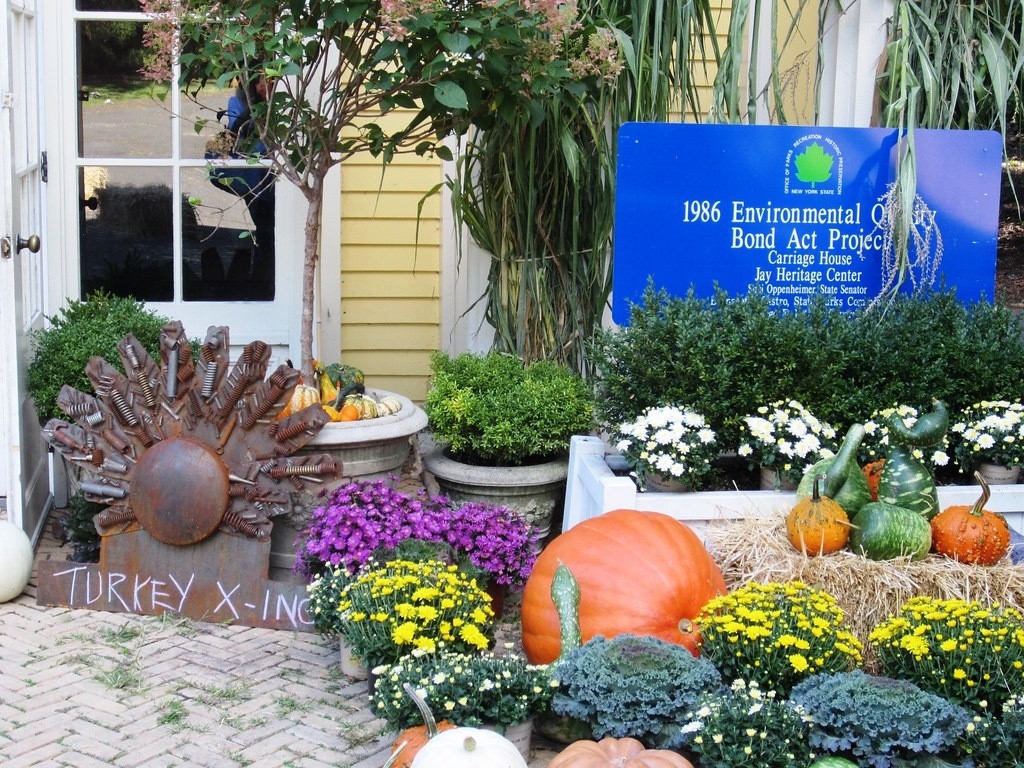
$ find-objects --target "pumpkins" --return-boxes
[388,683,693,768]
[785,459,1011,568]
[274,358,401,421]
[521,510,729,661]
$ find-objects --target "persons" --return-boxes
[227,52,274,232]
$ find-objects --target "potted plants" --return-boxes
[137,0,428,568]
[416,351,600,624]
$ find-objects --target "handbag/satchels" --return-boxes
[204,130,270,197]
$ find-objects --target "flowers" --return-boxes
[294,400,1024,768]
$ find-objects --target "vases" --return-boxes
[480,721,533,763]
[760,467,798,490]
[337,629,369,681]
[977,463,1021,484]
[644,470,695,493]
